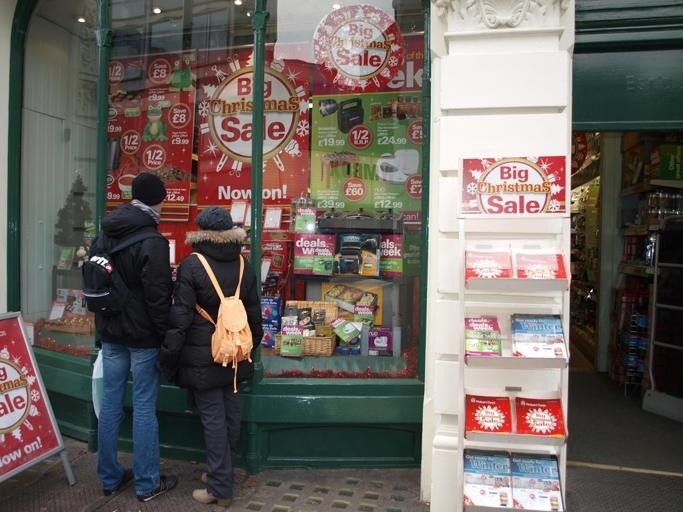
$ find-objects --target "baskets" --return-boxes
[274,299,339,358]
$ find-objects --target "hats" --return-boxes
[130,173,168,207]
[194,206,234,229]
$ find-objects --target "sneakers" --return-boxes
[136,474,179,502]
[102,469,135,496]
[191,471,234,507]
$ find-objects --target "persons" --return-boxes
[86,173,178,501]
[158,205,262,506]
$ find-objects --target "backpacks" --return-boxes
[188,249,253,366]
[80,229,164,319]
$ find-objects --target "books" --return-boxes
[464,242,564,512]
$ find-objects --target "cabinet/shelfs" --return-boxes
[618,177,682,279]
